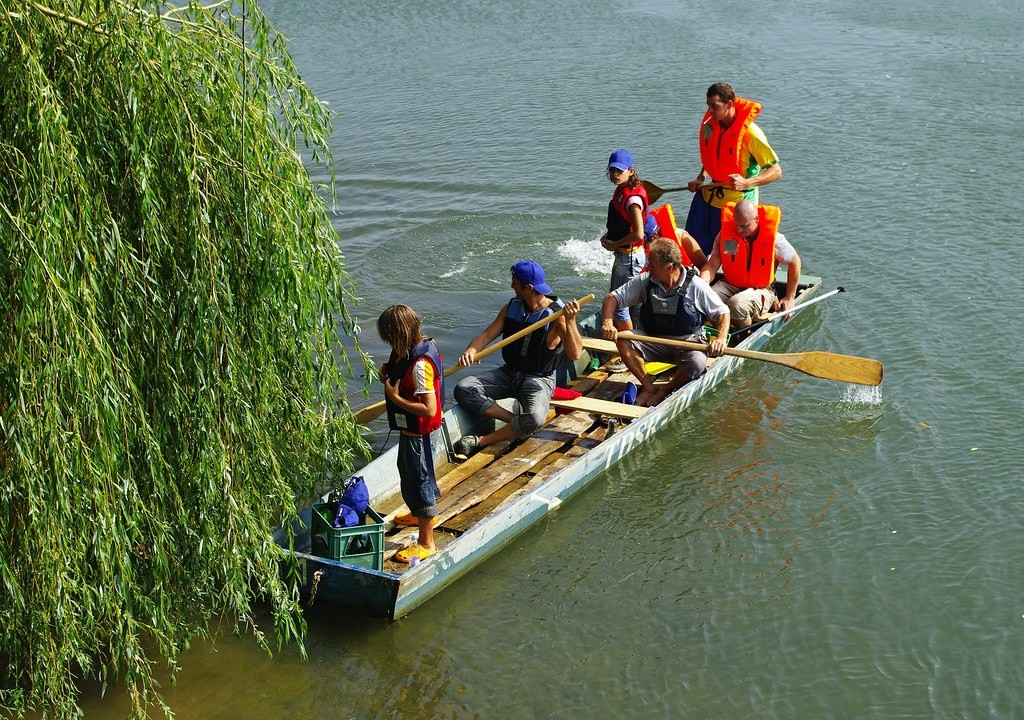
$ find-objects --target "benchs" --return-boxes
[581,336,718,368]
[550,395,650,420]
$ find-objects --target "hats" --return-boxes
[510,260,553,295]
[606,149,633,171]
[644,214,658,243]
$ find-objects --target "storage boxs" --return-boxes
[311,501,385,571]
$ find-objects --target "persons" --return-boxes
[460,260,582,454]
[685,82,782,259]
[601,238,730,406]
[700,200,801,348]
[600,150,648,372]
[641,204,708,276]
[376,304,445,563]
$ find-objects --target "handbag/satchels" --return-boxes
[334,476,369,528]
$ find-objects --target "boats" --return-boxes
[273,267,824,622]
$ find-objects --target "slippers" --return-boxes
[395,544,438,562]
[460,435,479,455]
[394,513,438,526]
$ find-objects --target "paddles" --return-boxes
[700,284,849,348]
[616,332,883,388]
[638,178,736,207]
[344,292,596,427]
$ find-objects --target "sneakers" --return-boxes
[607,358,630,373]
